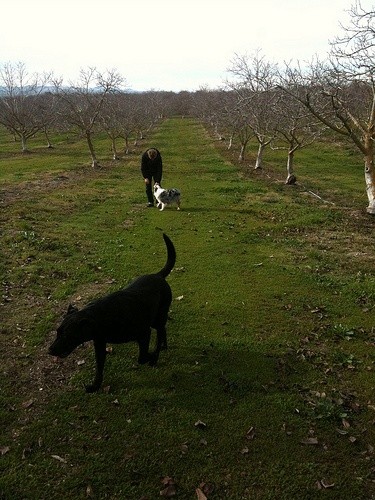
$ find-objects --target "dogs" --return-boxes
[46,233,176,393]
[153,184,181,211]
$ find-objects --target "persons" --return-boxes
[141,148,162,207]
